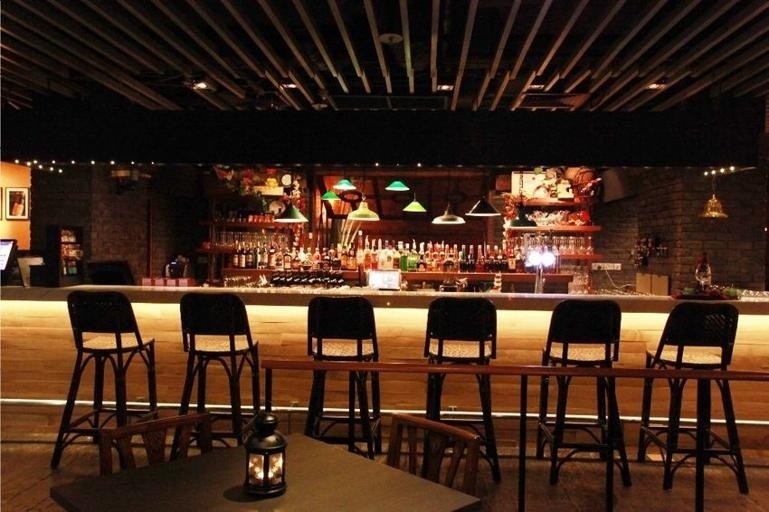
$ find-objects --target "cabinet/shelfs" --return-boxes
[217,265,574,294]
[210,193,314,285]
[44,222,84,285]
[503,195,603,268]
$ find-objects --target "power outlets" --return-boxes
[600,262,608,271]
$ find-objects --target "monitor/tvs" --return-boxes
[0,239,17,272]
[368,269,401,290]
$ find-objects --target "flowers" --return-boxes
[216,166,269,213]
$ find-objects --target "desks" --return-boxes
[47,428,483,511]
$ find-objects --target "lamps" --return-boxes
[240,408,287,496]
[698,168,732,224]
[270,167,543,228]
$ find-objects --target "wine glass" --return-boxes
[522,231,595,256]
[695,263,712,292]
[215,230,287,248]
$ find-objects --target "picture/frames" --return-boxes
[4,185,31,222]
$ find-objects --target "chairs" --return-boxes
[168,290,266,469]
[420,292,507,488]
[635,299,757,511]
[85,407,217,471]
[295,291,385,462]
[89,257,137,285]
[50,287,159,476]
[381,408,484,493]
[535,299,628,493]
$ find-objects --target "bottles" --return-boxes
[270,265,346,287]
[233,240,323,271]
[323,230,515,273]
